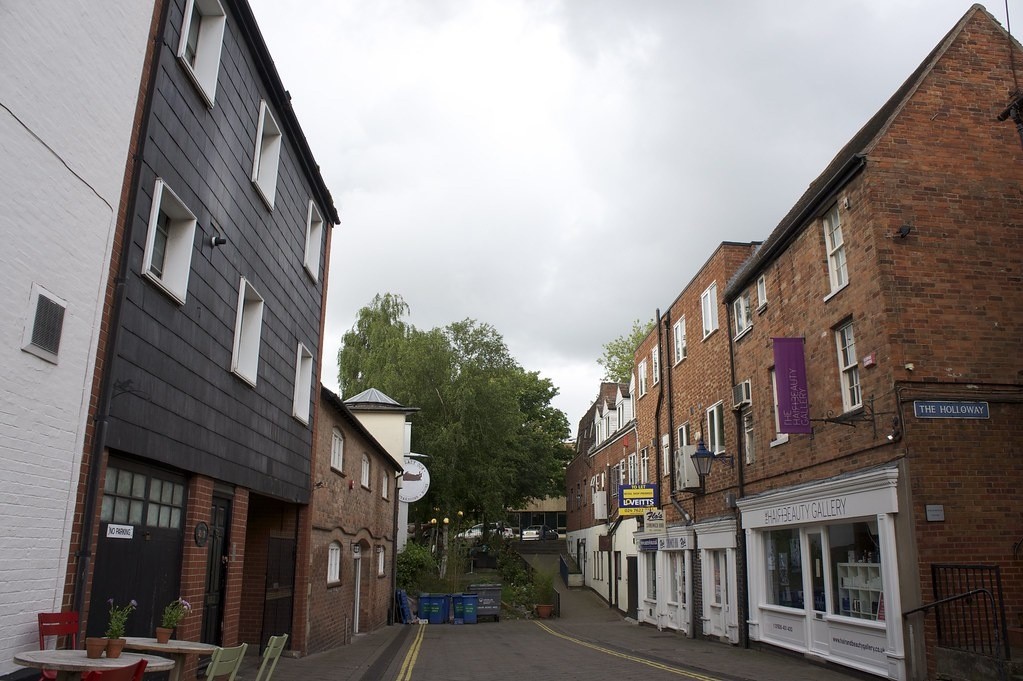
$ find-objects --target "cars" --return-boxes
[522,525,560,540]
[453,523,514,540]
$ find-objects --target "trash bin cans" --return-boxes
[470,583,502,622]
[462,593,478,623]
[429,594,449,624]
[417,593,430,619]
[451,593,464,624]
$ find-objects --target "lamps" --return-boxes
[690,436,734,476]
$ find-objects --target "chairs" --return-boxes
[206,633,289,681]
[37,611,79,681]
[84,658,148,681]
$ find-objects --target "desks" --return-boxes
[13,649,176,681]
[119,637,219,681]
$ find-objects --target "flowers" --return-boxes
[106,598,137,638]
[160,596,192,628]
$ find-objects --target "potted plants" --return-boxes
[534,555,559,618]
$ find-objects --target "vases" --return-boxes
[106,639,126,657]
[155,627,174,644]
[86,638,109,658]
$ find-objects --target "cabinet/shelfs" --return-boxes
[837,562,882,620]
